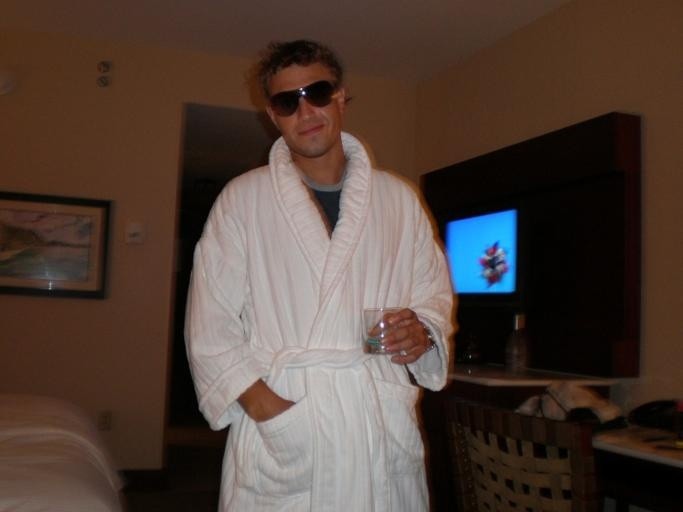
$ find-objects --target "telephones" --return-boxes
[628,398,683,427]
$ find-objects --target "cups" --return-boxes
[362,307,402,356]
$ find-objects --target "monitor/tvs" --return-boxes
[444,207,520,309]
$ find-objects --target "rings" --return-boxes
[398,348,408,358]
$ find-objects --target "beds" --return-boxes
[0,386,122,510]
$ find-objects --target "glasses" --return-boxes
[267,81,340,116]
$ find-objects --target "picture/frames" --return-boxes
[0,191,115,302]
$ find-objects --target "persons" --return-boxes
[184,41,456,511]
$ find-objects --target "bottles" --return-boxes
[507,312,531,377]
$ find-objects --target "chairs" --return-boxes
[445,392,600,512]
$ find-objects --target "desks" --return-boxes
[447,365,683,476]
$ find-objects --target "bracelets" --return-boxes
[424,325,436,354]
